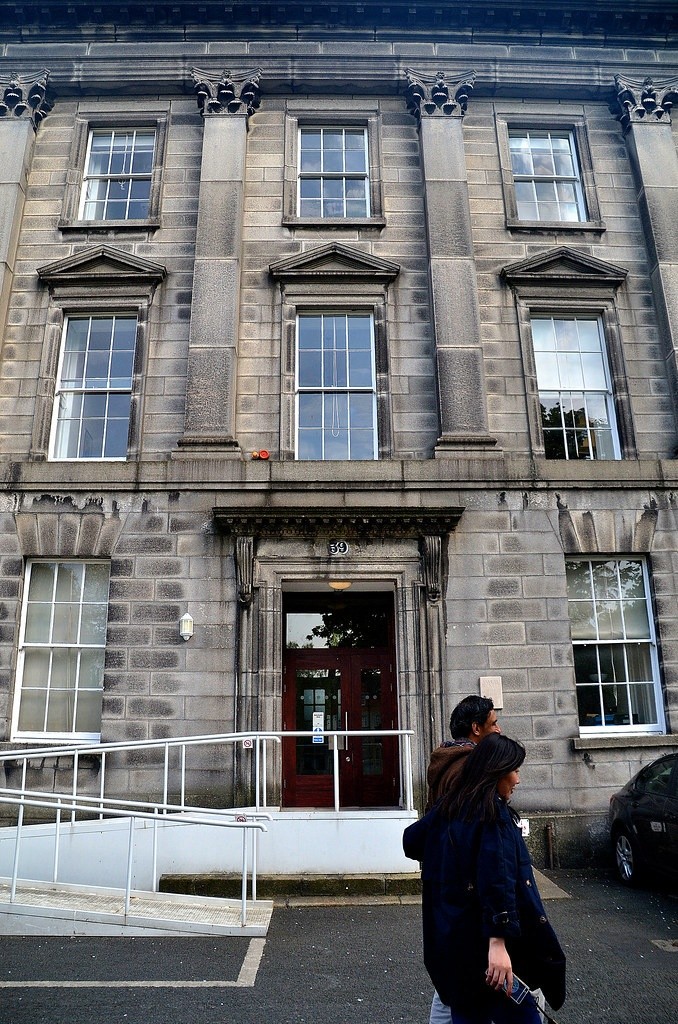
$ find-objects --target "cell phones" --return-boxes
[486,967,530,1005]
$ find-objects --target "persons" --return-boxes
[402,697,566,1024]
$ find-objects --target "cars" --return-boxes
[608,752,678,889]
